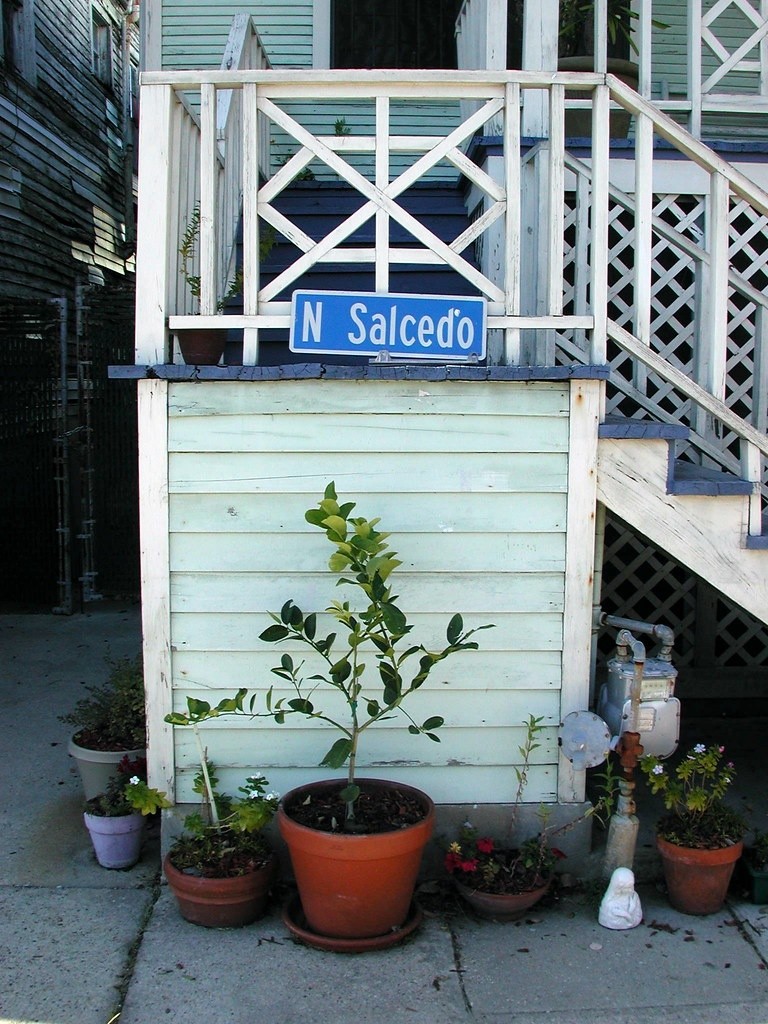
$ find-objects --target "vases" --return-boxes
[655,827,745,915]
[448,869,554,915]
[163,849,282,930]
[84,795,147,870]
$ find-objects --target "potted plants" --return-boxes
[176,199,279,364]
[558,0,671,139]
[736,826,768,905]
[164,481,499,936]
[54,650,146,800]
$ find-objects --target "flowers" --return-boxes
[641,742,752,846]
[126,744,282,875]
[445,711,623,896]
[76,753,147,816]
